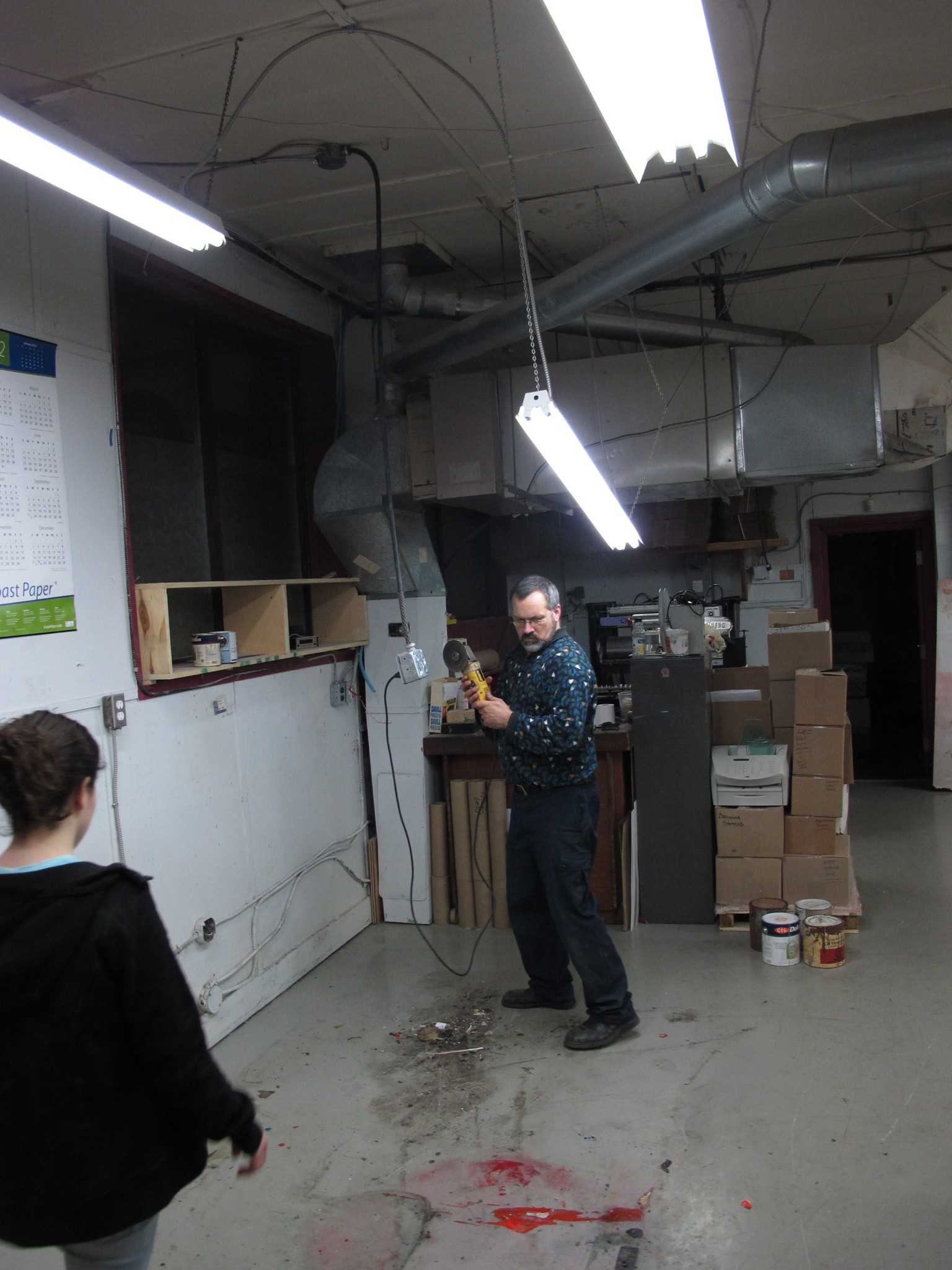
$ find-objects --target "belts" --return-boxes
[514,772,596,795]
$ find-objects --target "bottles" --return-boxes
[631,618,646,658]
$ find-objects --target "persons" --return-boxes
[0,710,268,1270]
[460,576,640,1050]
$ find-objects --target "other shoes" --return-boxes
[501,985,576,1010]
[565,1014,640,1049]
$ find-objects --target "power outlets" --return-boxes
[102,694,127,731]
[330,681,347,706]
[396,648,428,683]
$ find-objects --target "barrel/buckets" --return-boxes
[664,629,689,656]
[749,898,789,952]
[794,898,832,934]
[761,912,801,966]
[802,914,845,968]
[190,630,238,667]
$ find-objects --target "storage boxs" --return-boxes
[710,608,852,909]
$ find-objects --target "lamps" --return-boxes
[0,34,244,253]
[487,0,668,552]
[542,0,739,183]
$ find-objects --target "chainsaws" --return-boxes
[442,639,492,705]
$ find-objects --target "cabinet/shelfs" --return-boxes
[134,576,371,680]
[630,654,718,923]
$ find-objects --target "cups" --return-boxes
[617,691,632,722]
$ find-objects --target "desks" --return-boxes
[423,723,631,918]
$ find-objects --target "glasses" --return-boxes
[512,610,553,627]
